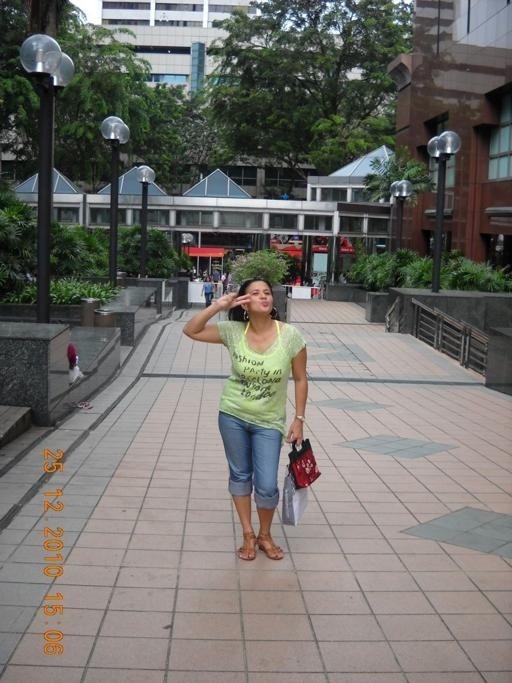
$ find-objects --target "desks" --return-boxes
[100,116,130,289]
[427,130,461,292]
[135,165,156,277]
[390,180,413,251]
[182,233,193,256]
[19,33,76,322]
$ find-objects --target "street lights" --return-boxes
[282,441,308,526]
[288,439,322,490]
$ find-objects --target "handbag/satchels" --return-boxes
[294,415,305,424]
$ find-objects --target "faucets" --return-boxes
[237,530,257,560]
[257,528,285,560]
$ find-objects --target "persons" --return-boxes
[182,277,309,561]
[311,269,351,287]
[61,324,85,385]
[190,266,230,308]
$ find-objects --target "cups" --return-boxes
[240,276,264,293]
